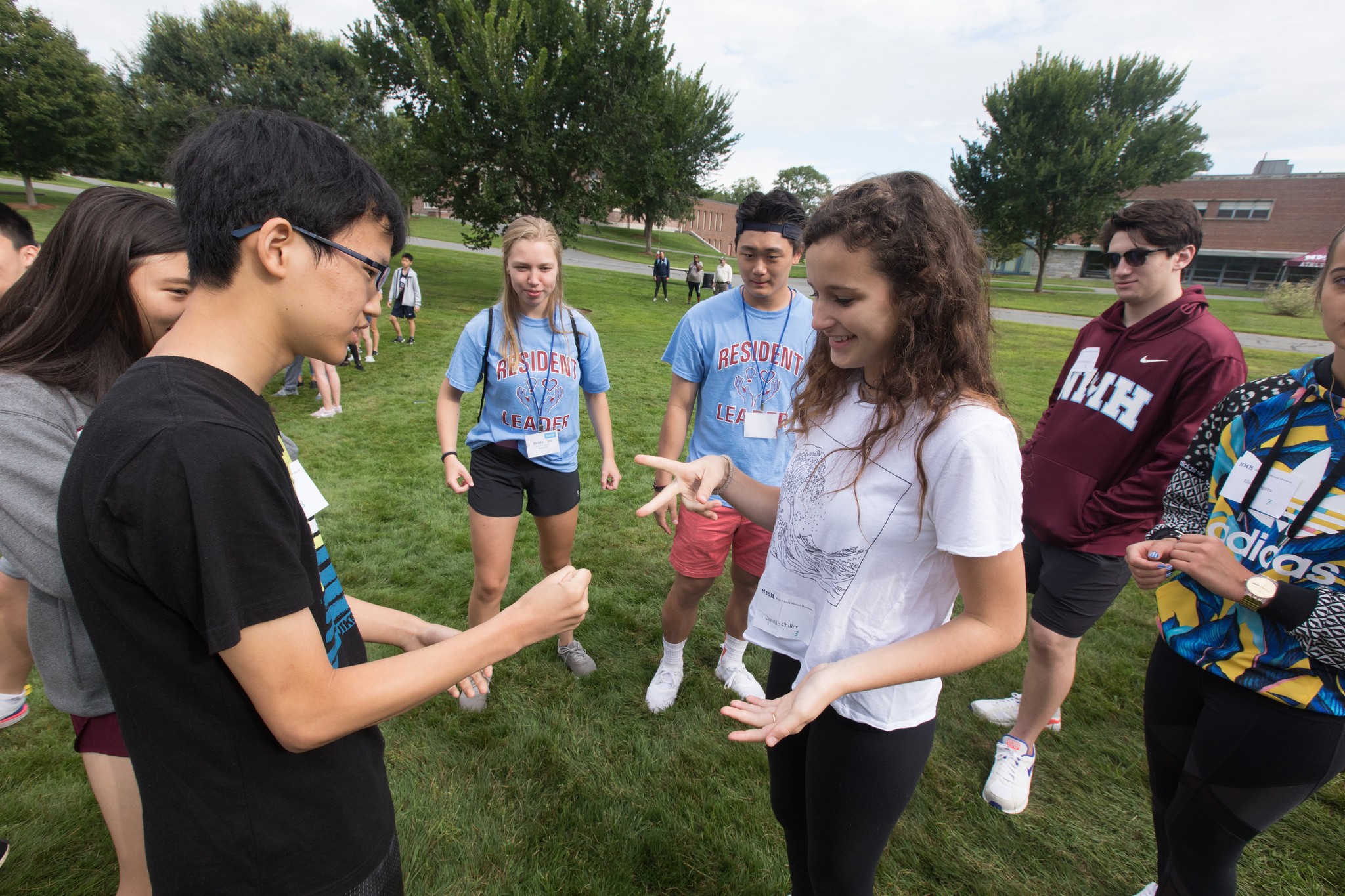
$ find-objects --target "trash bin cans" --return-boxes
[702,272,714,289]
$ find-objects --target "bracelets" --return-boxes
[711,454,734,495]
[441,451,458,463]
[653,482,668,492]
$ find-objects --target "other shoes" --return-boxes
[296,380,305,387]
[0,683,33,729]
[310,381,318,389]
[1133,880,1159,896]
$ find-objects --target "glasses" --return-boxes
[1097,248,1172,270]
[232,220,390,292]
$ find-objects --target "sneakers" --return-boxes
[270,387,298,397]
[333,404,343,415]
[457,672,488,712]
[644,659,684,714]
[714,643,766,703]
[980,729,1036,815]
[339,336,415,372]
[310,406,336,418]
[315,392,322,401]
[556,637,597,679]
[969,691,1061,735]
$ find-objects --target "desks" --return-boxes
[648,265,704,274]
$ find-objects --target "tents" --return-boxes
[1274,246,1329,290]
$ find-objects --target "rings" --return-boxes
[772,713,777,723]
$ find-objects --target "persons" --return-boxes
[970,197,1248,815]
[653,251,670,302]
[1124,223,1345,896]
[0,187,193,895]
[712,257,732,296]
[437,215,621,714]
[57,109,593,896]
[272,254,422,419]
[686,255,703,304]
[636,171,1028,896]
[644,187,821,713]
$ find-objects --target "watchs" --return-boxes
[696,262,698,264]
[1238,573,1278,613]
